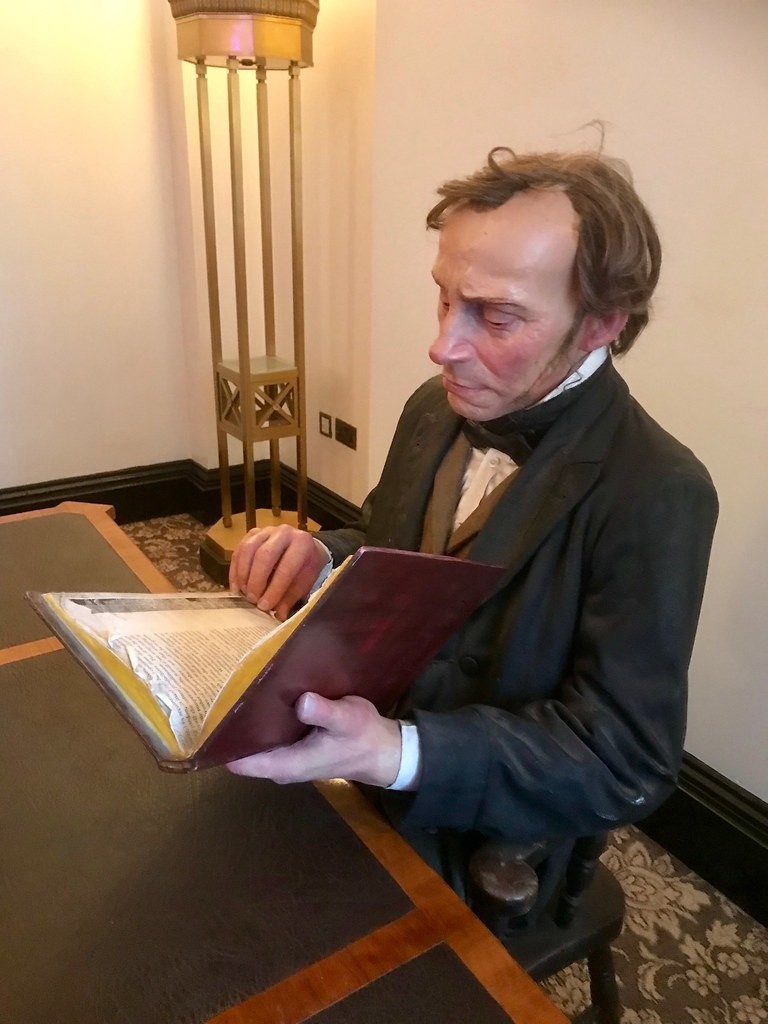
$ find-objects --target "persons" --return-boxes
[227,147,720,938]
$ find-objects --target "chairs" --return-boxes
[457,834,627,1024]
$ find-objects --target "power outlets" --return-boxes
[319,411,333,439]
[335,417,357,451]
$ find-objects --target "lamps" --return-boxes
[169,0,322,594]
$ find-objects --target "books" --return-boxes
[23,548,506,773]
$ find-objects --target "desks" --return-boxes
[0,500,573,1024]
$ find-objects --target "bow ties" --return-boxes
[459,406,540,470]
